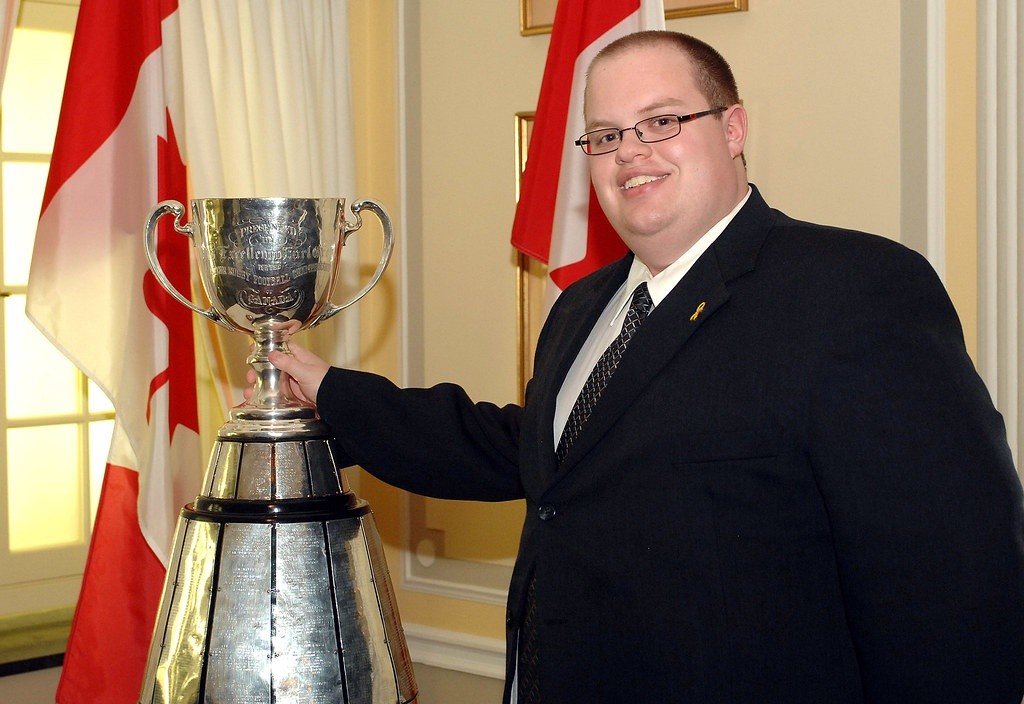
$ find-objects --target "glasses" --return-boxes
[574,107,729,156]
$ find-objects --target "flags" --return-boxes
[510,1,666,291]
[24,2,198,704]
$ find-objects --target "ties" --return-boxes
[517,282,653,704]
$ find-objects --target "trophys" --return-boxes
[143,198,396,437]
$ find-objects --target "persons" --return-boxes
[244,31,1024,703]
